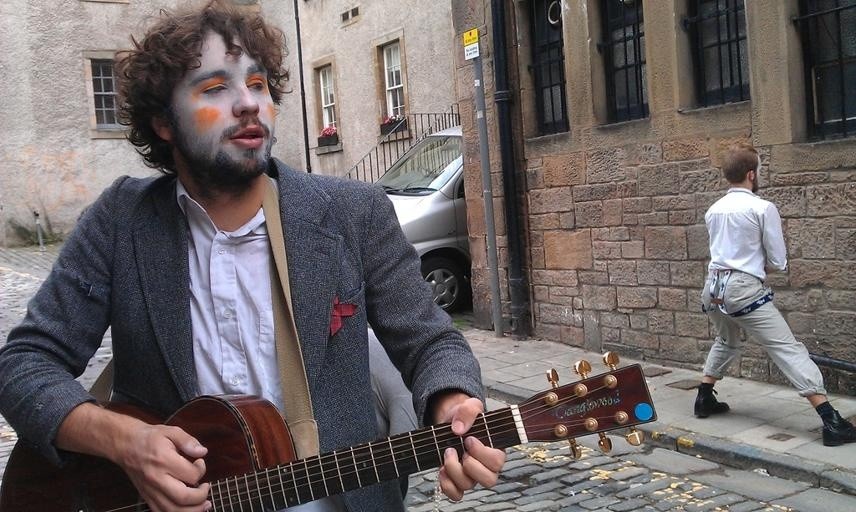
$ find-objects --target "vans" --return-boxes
[372,123,472,317]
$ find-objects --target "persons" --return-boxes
[692,147,856,446]
[0,5,506,511]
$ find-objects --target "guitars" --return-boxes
[0,350,657,512]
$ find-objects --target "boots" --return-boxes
[821,410,855,447]
[692,383,730,418]
